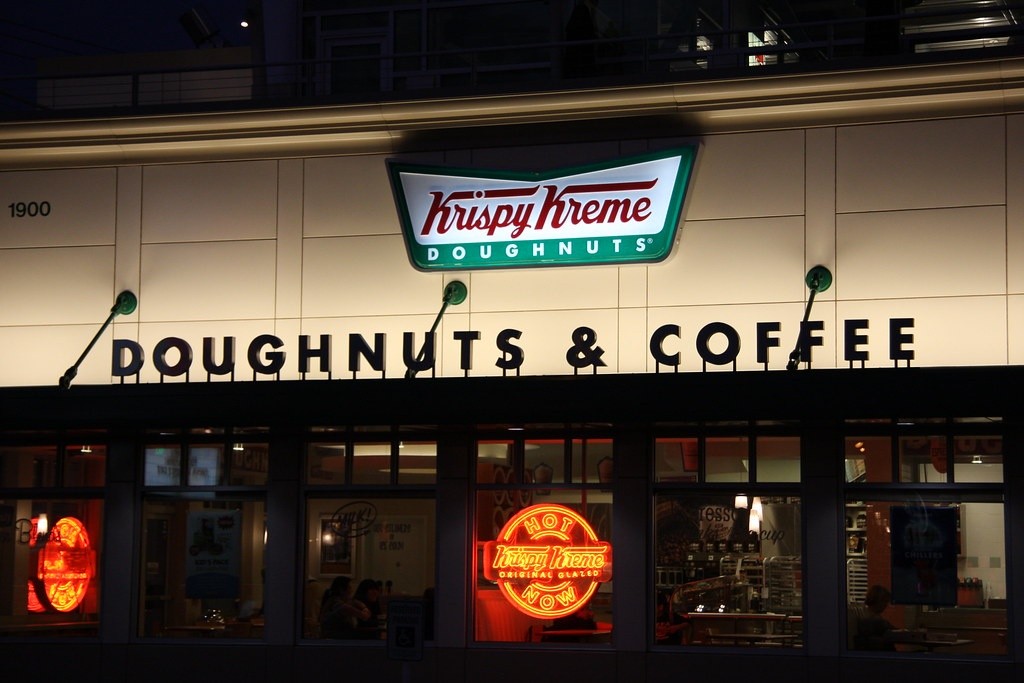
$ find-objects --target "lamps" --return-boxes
[786,265,832,372]
[59,291,137,391]
[404,281,467,380]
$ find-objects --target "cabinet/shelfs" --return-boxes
[846,504,867,556]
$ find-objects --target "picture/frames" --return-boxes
[316,514,356,579]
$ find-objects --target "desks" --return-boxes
[684,612,804,648]
[537,630,612,645]
[165,625,233,639]
[869,636,975,654]
[710,633,799,649]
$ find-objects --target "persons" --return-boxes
[318,576,395,642]
[847,585,896,652]
